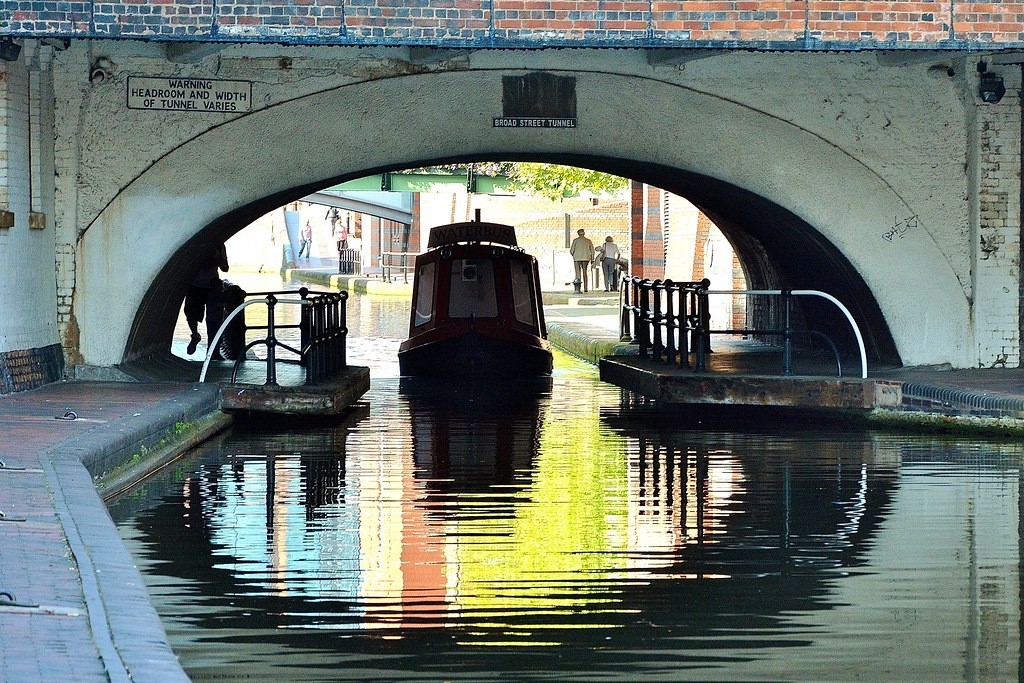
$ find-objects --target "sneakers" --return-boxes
[186,332,201,355]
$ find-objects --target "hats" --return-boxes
[577,228,584,232]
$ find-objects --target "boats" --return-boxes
[396,220,554,377]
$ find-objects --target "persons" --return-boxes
[570,227,594,292]
[334,218,349,256]
[297,218,313,262]
[183,238,231,361]
[324,206,340,237]
[599,236,620,293]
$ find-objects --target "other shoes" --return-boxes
[306,257,309,262]
[298,253,301,257]
